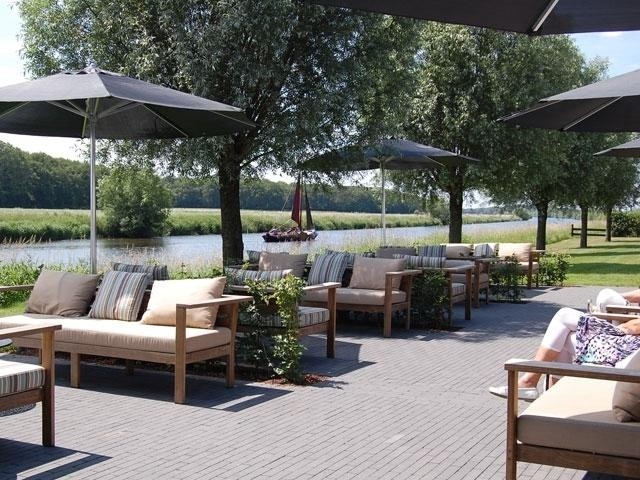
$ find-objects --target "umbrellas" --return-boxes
[294,137,481,248]
[308,0,640,38]
[593,138,640,158]
[495,68,640,133]
[1,63,259,275]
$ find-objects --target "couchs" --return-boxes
[225,281,342,376]
[1,285,254,404]
[300,265,424,337]
[0,322,62,446]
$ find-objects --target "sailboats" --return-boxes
[263,172,318,242]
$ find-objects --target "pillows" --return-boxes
[325,243,532,266]
[25,270,98,318]
[110,261,170,280]
[349,255,407,290]
[139,275,228,329]
[87,269,152,321]
[246,249,289,262]
[259,251,308,281]
[224,268,293,314]
[612,350,640,424]
[307,253,348,285]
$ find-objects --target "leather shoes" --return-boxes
[587,299,592,313]
[488,386,539,402]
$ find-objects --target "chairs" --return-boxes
[504,303,640,480]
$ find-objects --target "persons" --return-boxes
[488,287,640,403]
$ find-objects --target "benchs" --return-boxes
[304,250,544,327]
